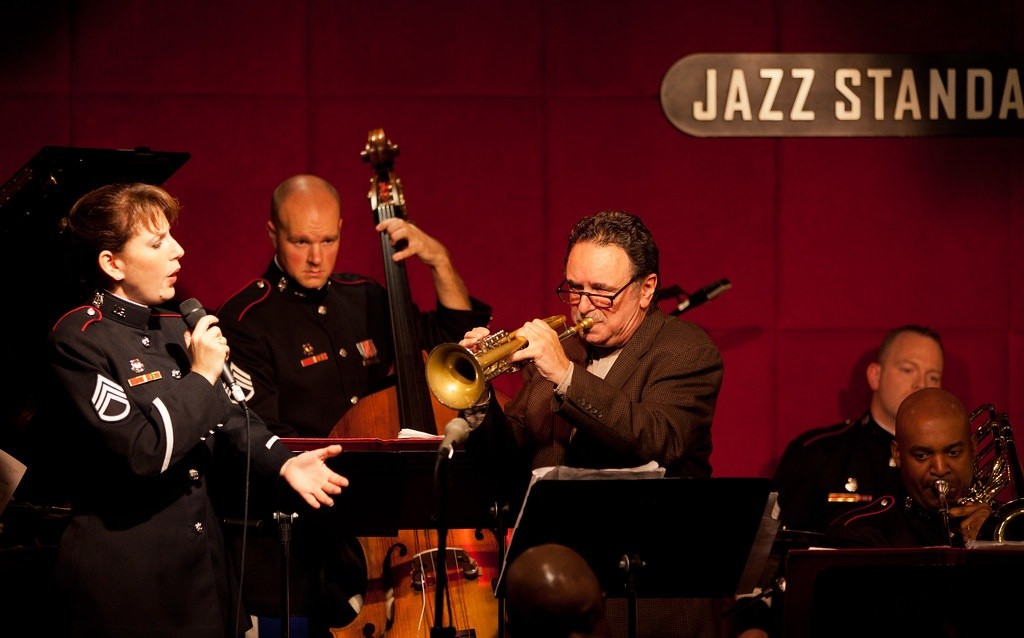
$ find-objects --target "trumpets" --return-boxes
[424,310,593,410]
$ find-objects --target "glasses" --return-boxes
[556,273,643,309]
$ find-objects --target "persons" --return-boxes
[811,388,1024,638]
[36,183,349,638]
[456,212,723,638]
[732,324,945,638]
[201,169,496,638]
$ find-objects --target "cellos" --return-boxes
[328,127,503,638]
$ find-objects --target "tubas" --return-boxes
[932,403,1024,550]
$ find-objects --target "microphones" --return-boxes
[179,298,248,411]
[668,279,732,316]
